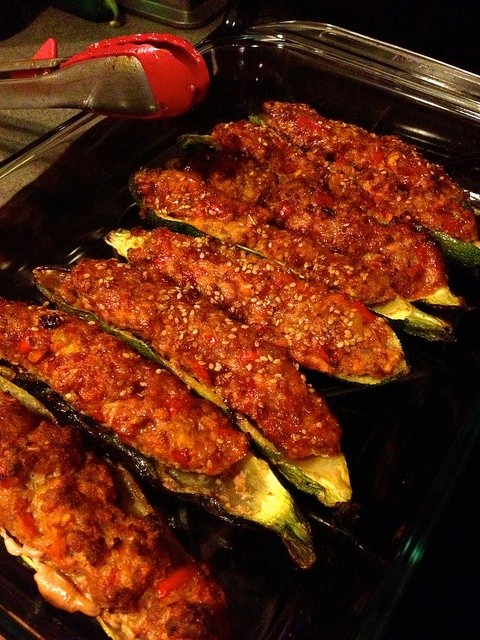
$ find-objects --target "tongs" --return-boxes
[0,32,210,122]
